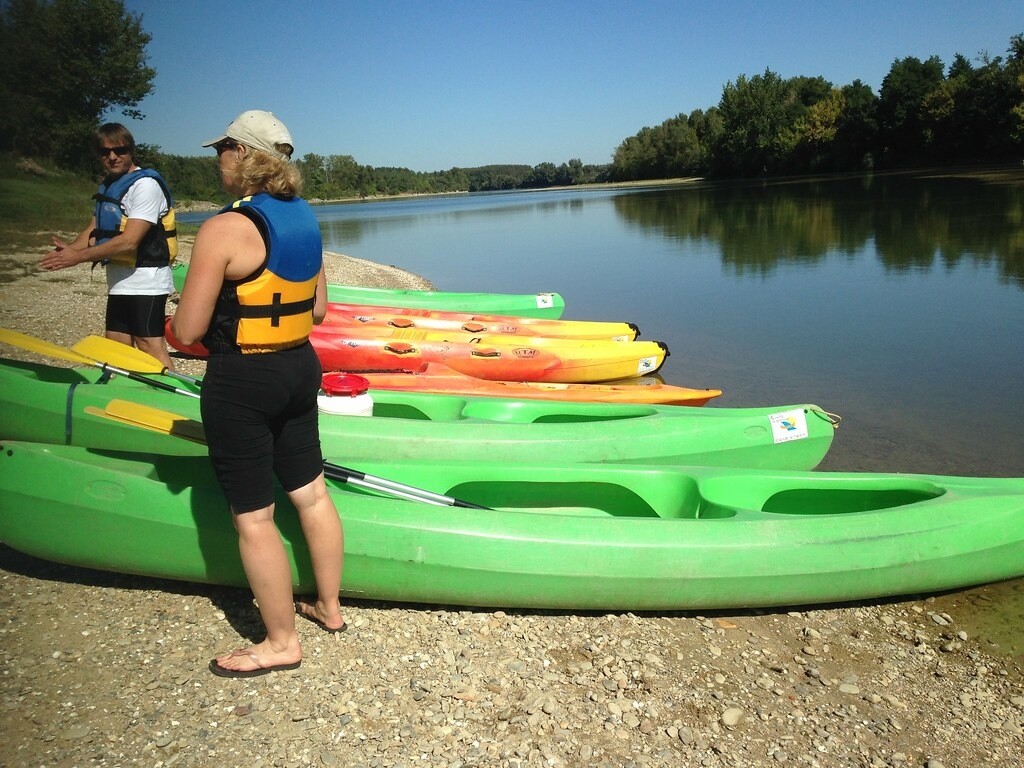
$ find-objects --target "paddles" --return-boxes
[82,406,461,506]
[72,334,202,389]
[106,399,496,510]
[1,325,204,399]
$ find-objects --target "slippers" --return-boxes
[295,597,348,634]
[208,649,301,678]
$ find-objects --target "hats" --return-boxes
[203,109,294,164]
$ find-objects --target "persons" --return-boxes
[170,109,347,679]
[39,122,178,371]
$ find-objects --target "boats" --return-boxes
[320,302,640,339]
[326,373,721,406]
[0,440,1022,612]
[324,282,567,320]
[308,327,672,383]
[1,360,836,471]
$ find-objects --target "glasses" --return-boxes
[99,146,133,155]
[216,144,242,156]
